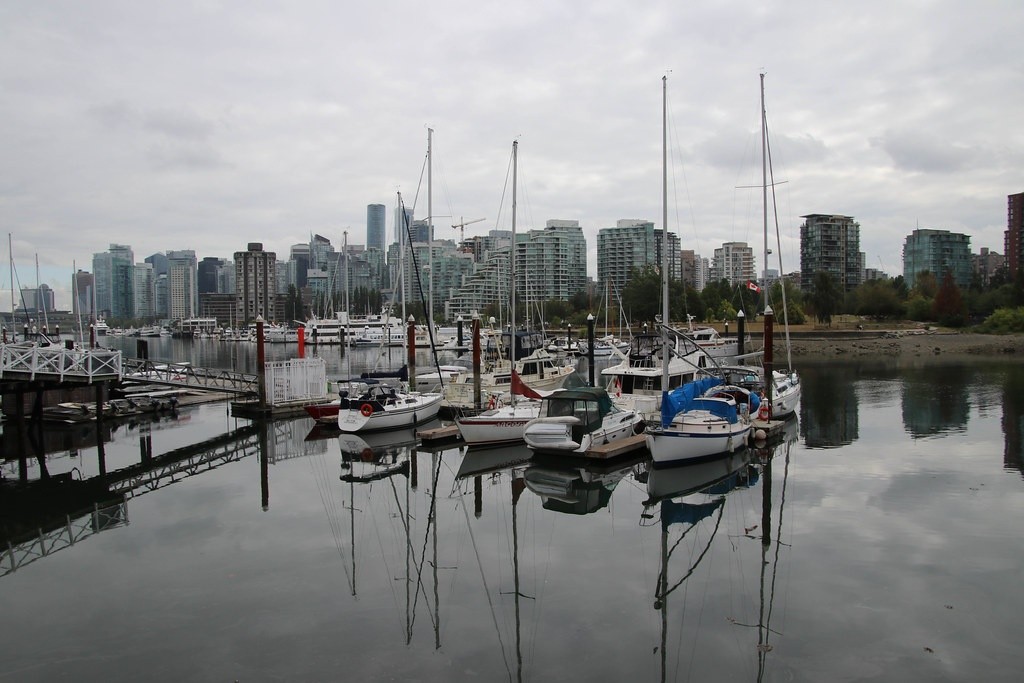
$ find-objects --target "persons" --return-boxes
[488,394,496,409]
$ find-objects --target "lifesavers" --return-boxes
[759,406,773,421]
[361,403,373,417]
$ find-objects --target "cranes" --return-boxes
[452,216,487,247]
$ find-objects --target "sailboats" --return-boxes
[0,65,804,471]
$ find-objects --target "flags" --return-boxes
[747,279,760,293]
[510,369,542,399]
